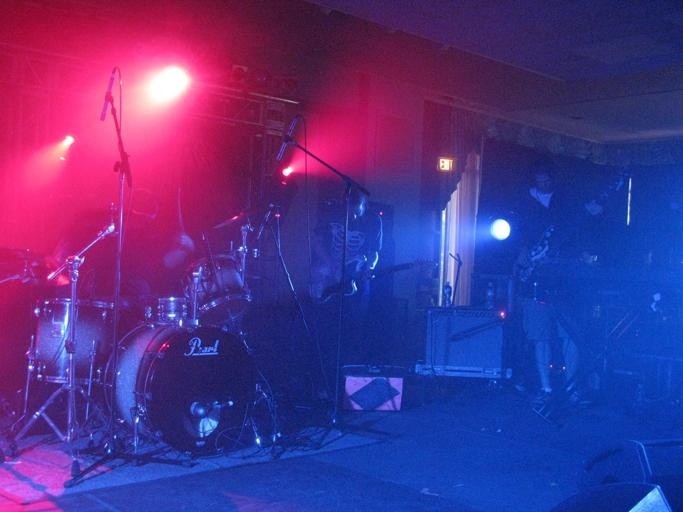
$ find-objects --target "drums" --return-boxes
[35,298,129,389]
[157,296,189,327]
[179,253,252,326]
[103,321,254,456]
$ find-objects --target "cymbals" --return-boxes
[211,204,268,230]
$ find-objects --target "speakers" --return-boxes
[555,478,675,512]
[423,307,510,379]
[603,434,680,483]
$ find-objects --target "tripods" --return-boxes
[5,240,116,477]
[294,142,390,454]
[68,98,196,486]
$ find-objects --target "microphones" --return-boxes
[248,204,276,251]
[277,121,291,164]
[99,76,115,123]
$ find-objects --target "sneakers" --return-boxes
[529,387,593,411]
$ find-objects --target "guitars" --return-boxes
[310,253,438,302]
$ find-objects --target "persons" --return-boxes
[312,189,386,367]
[498,162,612,412]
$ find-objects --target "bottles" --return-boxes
[484,281,494,311]
[442,281,452,309]
